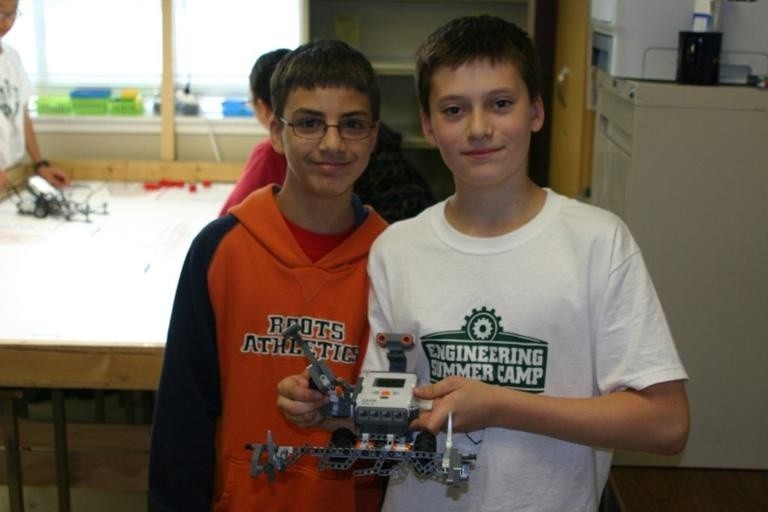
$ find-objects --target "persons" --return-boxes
[0,0,70,201]
[219,50,291,218]
[278,14,690,512]
[150,39,391,512]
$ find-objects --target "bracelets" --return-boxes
[33,159,50,169]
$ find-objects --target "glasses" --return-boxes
[277,116,379,141]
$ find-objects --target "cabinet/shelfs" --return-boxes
[301,0,538,209]
